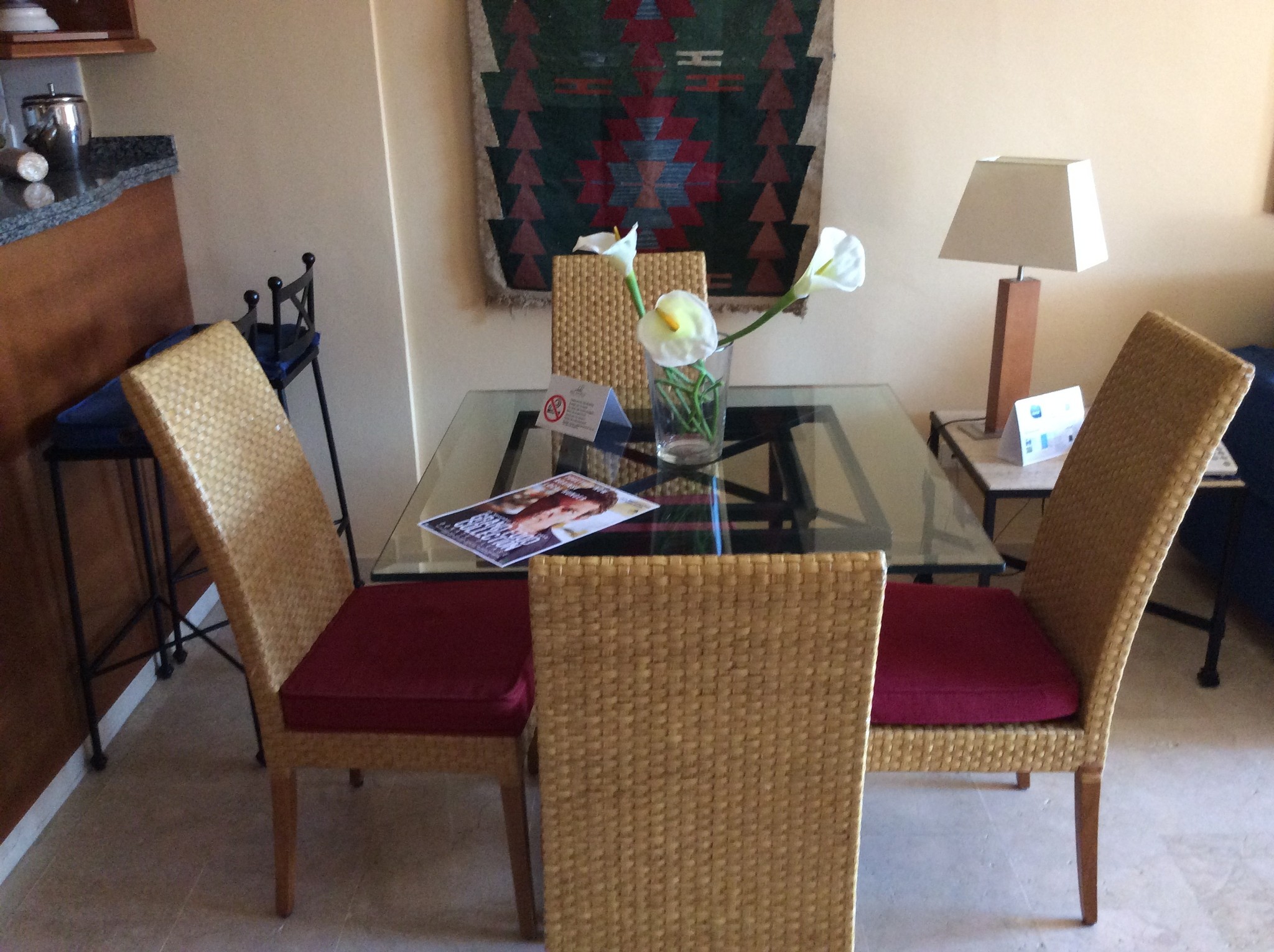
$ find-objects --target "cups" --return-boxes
[0,146,49,182]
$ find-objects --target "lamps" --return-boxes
[943,155,1109,439]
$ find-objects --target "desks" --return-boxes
[927,408,1249,686]
[373,387,1010,587]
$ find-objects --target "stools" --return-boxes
[40,255,359,771]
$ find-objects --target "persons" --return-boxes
[430,486,618,565]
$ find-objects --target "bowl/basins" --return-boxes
[1,8,60,33]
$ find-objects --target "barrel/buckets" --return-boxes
[22,83,91,160]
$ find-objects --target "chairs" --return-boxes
[552,250,733,531]
[863,308,1256,924]
[131,316,540,937]
[519,544,893,952]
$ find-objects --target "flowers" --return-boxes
[572,222,866,439]
